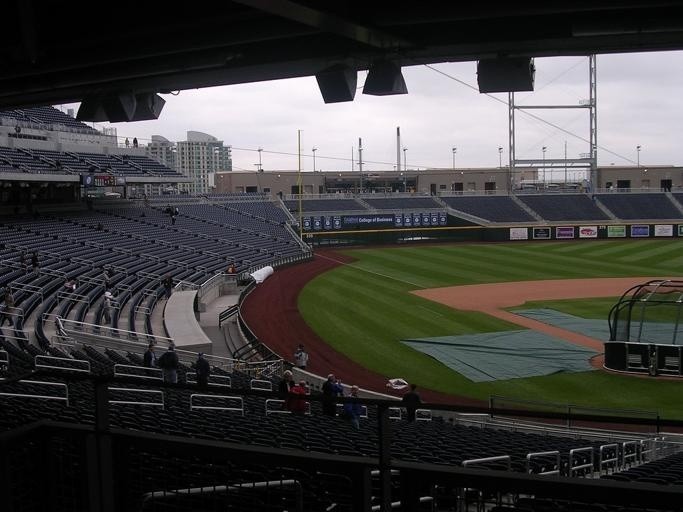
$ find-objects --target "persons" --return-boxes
[125,138,130,148]
[56,158,62,169]
[279,190,282,200]
[166,203,179,225]
[133,138,138,149]
[0,240,421,430]
